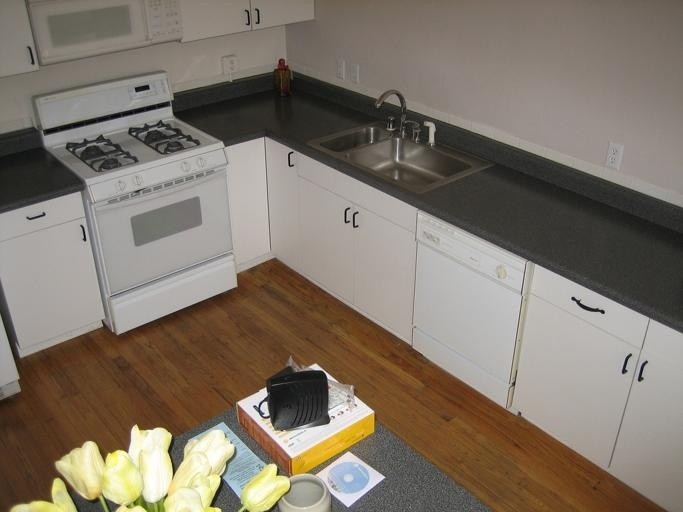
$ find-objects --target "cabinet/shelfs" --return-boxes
[176,0,315,44]
[0,0,40,79]
[0,192,105,359]
[299,176,413,347]
[265,138,299,275]
[511,264,683,512]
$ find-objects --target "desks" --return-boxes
[47,407,495,512]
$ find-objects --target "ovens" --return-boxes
[84,141,238,337]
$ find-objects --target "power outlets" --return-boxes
[605,141,624,170]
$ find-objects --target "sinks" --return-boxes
[305,122,401,155]
[345,132,496,194]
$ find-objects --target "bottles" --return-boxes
[273,58,291,97]
[276,473,332,512]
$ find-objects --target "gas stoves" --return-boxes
[53,117,222,179]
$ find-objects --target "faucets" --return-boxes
[423,121,436,145]
[374,88,407,129]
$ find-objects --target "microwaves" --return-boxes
[27,0,183,67]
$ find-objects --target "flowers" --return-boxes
[9,424,291,512]
